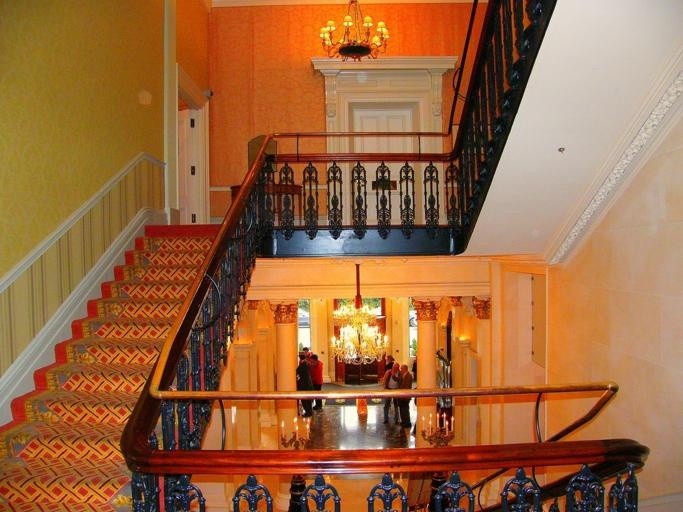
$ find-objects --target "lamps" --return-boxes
[319,0,390,63]
[329,263,389,367]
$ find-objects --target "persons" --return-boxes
[310,354,323,410]
[384,354,394,368]
[380,362,402,424]
[297,351,314,417]
[397,364,412,427]
[298,347,312,364]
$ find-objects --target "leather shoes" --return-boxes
[303,406,321,417]
[383,420,401,425]
